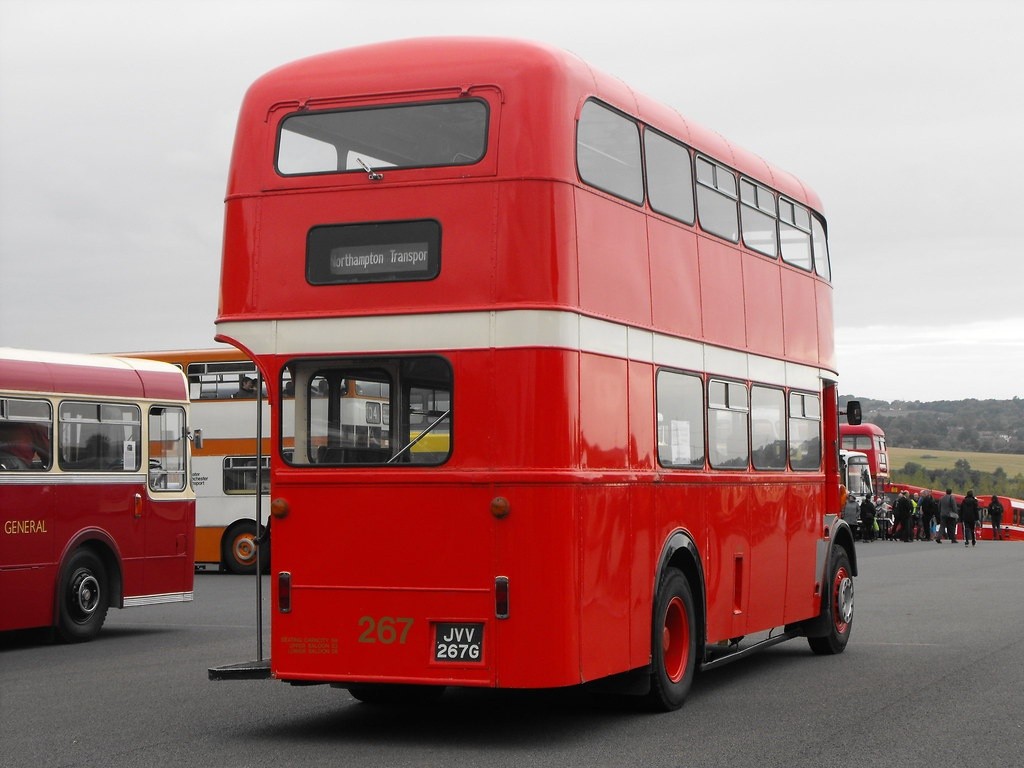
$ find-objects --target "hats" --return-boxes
[850,493,856,500]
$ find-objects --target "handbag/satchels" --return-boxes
[866,513,872,518]
[930,518,937,533]
[873,517,879,532]
[948,512,958,524]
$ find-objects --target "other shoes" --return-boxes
[952,539,958,543]
[965,542,969,546]
[936,539,941,543]
[972,541,976,546]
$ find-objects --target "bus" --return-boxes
[409,390,452,466]
[839,423,1024,540]
[213,34,860,714]
[101,351,391,574]
[0,345,197,636]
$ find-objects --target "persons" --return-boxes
[0,420,51,472]
[283,382,294,398]
[842,487,980,547]
[235,377,259,399]
[986,495,1004,542]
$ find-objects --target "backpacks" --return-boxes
[992,503,1000,514]
[892,501,897,516]
[895,500,906,520]
